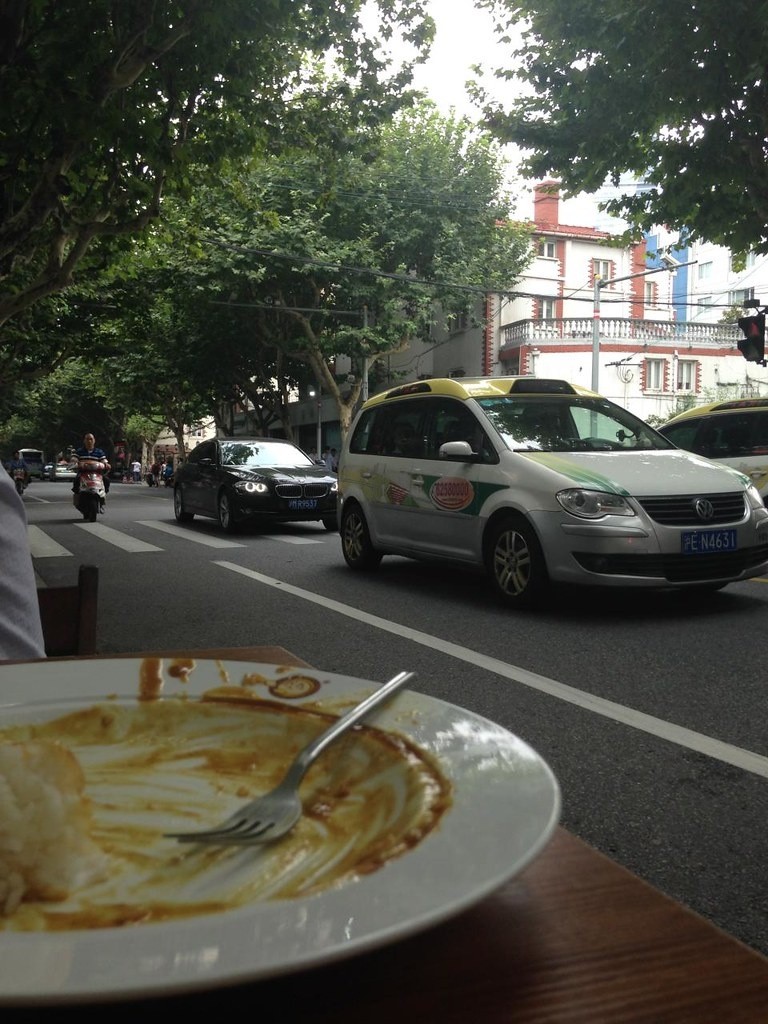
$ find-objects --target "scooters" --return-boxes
[68,448,111,521]
[7,464,30,496]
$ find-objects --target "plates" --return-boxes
[0,657,563,1009]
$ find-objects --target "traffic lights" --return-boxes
[735,313,767,363]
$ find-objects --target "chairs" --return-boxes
[37,563,98,657]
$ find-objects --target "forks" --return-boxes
[161,666,417,850]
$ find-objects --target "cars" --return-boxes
[52,462,78,482]
[335,374,768,608]
[173,437,341,536]
[43,465,53,479]
[614,393,768,512]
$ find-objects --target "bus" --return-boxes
[18,449,44,480]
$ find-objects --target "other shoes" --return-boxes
[100,507,105,514]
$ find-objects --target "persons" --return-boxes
[71,433,112,494]
[308,446,339,472]
[389,423,425,459]
[150,461,174,487]
[132,460,141,480]
[9,451,30,483]
[0,463,48,660]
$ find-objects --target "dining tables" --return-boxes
[0,645,768,1024]
